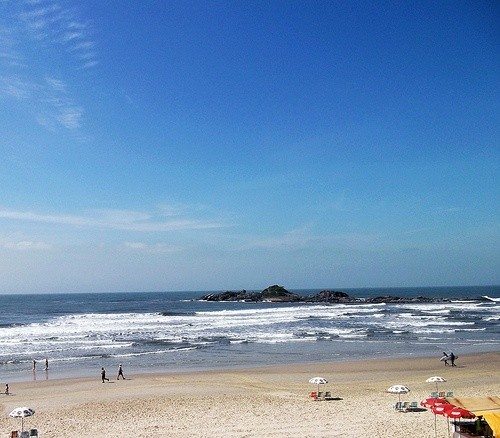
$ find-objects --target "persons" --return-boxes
[32,359,36,371]
[116,364,125,381]
[5,384,9,394]
[44,359,48,370]
[443,352,449,366]
[450,352,457,366]
[101,367,110,383]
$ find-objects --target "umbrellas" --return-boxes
[432,404,456,434]
[443,406,475,431]
[309,377,328,392]
[388,384,411,401]
[425,376,447,393]
[420,398,450,430]
[10,407,36,431]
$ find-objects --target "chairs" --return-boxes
[11,429,38,438]
[396,401,418,412]
[430,391,453,398]
[309,391,331,401]
